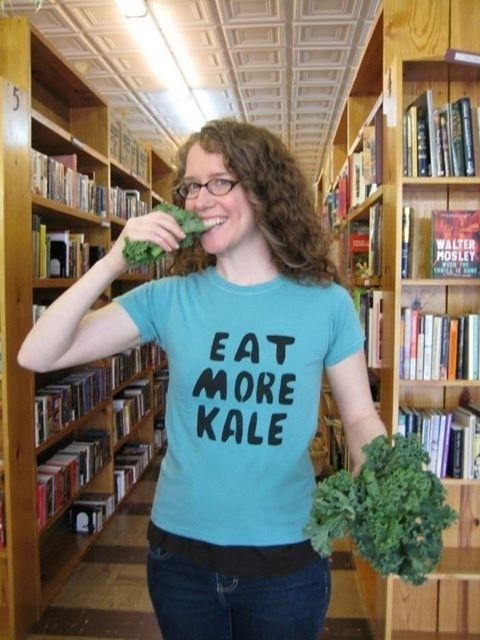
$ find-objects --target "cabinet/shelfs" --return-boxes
[0,13,179,640]
[316,1,480,640]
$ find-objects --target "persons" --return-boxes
[15,117,389,639]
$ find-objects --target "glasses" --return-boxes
[174,178,240,200]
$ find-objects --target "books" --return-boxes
[29,147,169,279]
[32,294,170,534]
[397,405,480,479]
[401,206,480,280]
[399,307,479,381]
[320,285,383,473]
[321,106,383,278]
[403,90,475,178]
